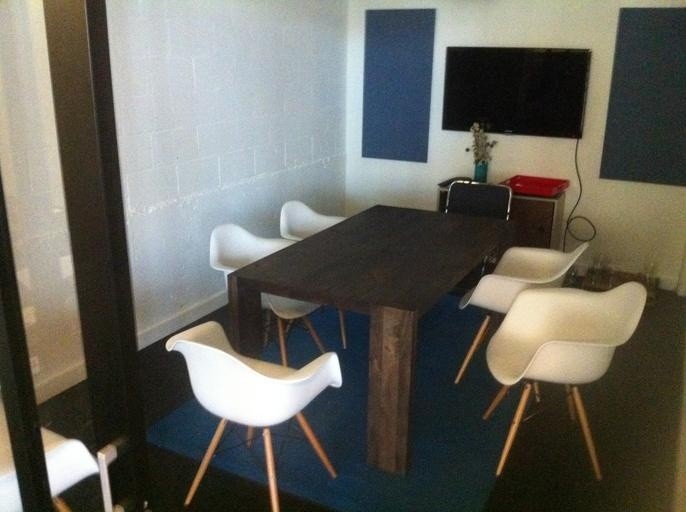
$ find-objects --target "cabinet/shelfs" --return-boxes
[437,175,566,296]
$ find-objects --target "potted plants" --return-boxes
[470,122,499,183]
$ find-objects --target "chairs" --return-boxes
[483,282,648,482]
[278,201,354,353]
[208,222,335,367]
[164,320,344,511]
[436,179,516,289]
[451,238,594,404]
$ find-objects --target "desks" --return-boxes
[0,402,101,511]
[227,204,521,473]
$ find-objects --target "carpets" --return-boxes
[144,282,684,510]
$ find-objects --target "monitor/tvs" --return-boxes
[443,47,592,139]
